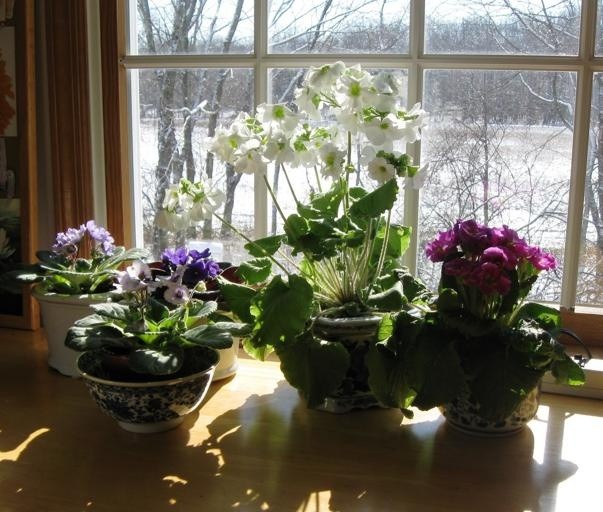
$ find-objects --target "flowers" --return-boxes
[374,218,586,425]
[6,219,150,295]
[152,61,430,420]
[146,246,247,314]
[64,259,254,378]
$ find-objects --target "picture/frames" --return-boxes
[0,0,42,331]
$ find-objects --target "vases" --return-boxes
[75,346,221,432]
[212,314,241,383]
[31,282,124,378]
[437,373,541,439]
[306,304,422,415]
